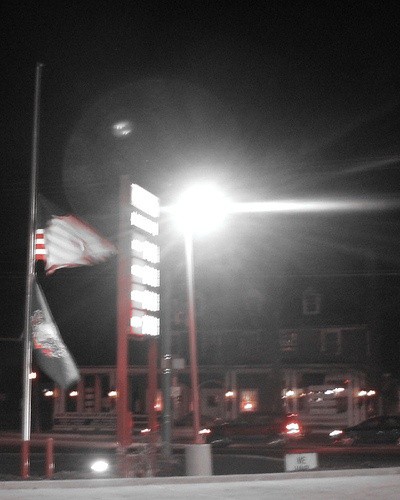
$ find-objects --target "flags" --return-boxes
[32,194,118,276]
[26,282,82,390]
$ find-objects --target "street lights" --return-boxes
[174,188,211,476]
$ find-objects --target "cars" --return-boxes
[199,411,304,449]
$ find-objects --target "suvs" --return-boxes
[330,414,400,448]
